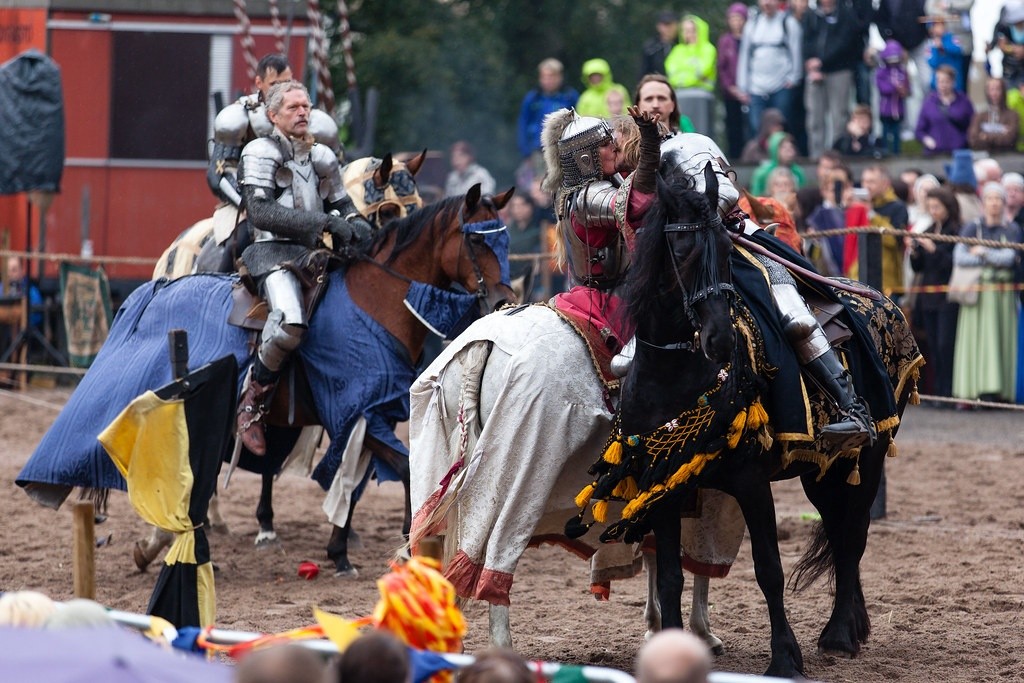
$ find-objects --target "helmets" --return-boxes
[540,106,615,191]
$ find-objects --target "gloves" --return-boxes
[324,212,374,268]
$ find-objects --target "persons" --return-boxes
[541,101,662,301]
[0,256,43,341]
[206,53,340,274]
[633,627,713,681]
[337,633,412,683]
[519,1,1024,414]
[234,80,376,457]
[444,140,496,202]
[233,642,324,683]
[459,646,535,683]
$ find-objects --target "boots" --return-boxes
[237,364,279,456]
[803,348,874,435]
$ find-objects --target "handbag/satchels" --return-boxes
[947,220,983,304]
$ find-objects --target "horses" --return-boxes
[617,159,910,683]
[133,148,428,570]
[79,182,515,573]
[438,188,801,648]
